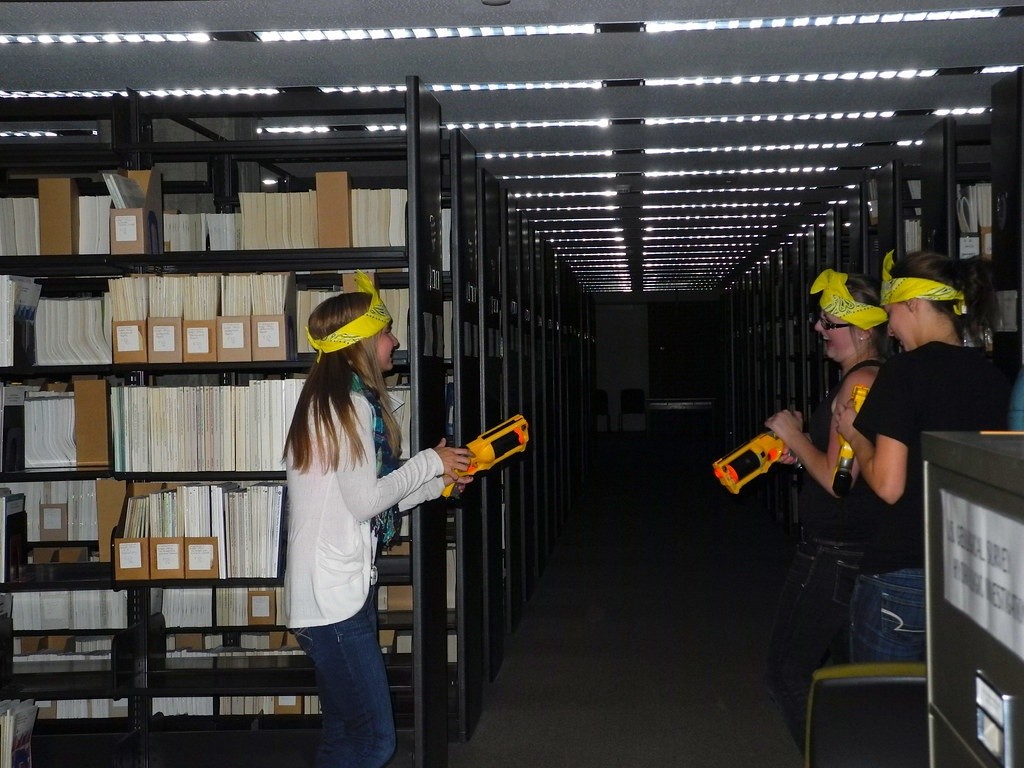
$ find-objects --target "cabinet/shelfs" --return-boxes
[732,67,1024,537]
[0,76,597,767]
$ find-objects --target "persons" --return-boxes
[839,253,1006,768]
[766,270,907,767]
[280,287,477,767]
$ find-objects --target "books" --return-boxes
[0,696,40,768]
[1,585,414,722]
[442,510,457,705]
[954,179,994,261]
[0,478,414,579]
[0,373,422,474]
[900,174,928,256]
[0,273,451,370]
[0,166,453,272]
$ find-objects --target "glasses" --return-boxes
[819,317,853,330]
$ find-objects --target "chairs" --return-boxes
[598,389,611,432]
[618,389,647,434]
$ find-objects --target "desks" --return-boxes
[645,397,716,442]
